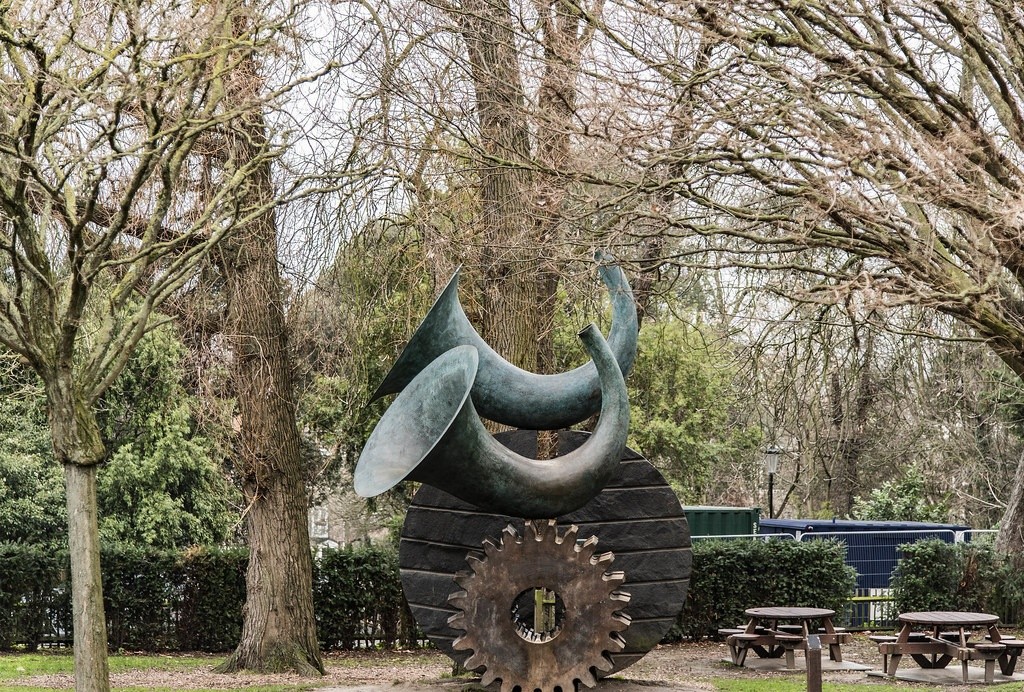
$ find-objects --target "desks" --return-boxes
[887,612,1022,684]
[730,606,843,668]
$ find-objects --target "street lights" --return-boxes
[764,446,782,519]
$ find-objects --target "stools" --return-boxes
[868,632,1024,676]
[717,625,851,667]
